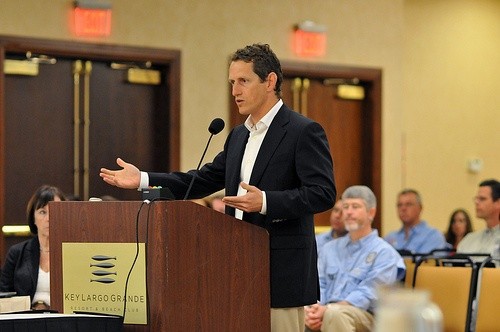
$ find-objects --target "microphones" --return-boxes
[183,118,225,200]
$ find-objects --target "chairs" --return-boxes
[397,248,500,332]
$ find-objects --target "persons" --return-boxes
[0,185,67,310]
[371,283,445,332]
[444,209,473,256]
[382,190,448,270]
[456,180,500,332]
[207,194,225,214]
[189,199,210,207]
[305,185,406,332]
[100,42,336,332]
[315,194,349,250]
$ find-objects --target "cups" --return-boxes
[371,284,443,332]
